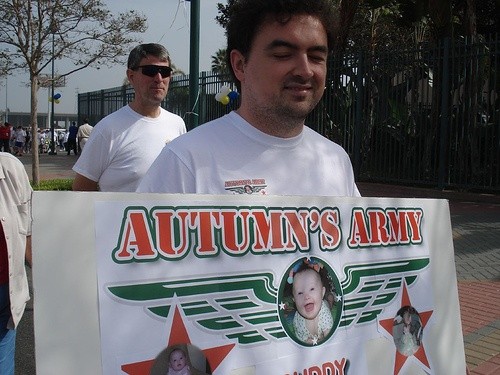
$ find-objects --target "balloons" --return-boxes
[215,86,237,109]
[48,94,61,104]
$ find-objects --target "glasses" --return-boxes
[130,65,173,78]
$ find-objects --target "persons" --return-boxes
[76,118,94,151]
[166,349,190,375]
[393,306,423,356]
[0,151,33,375]
[287,260,335,343]
[0,120,69,157]
[67,121,77,156]
[72,42,188,191]
[135,0,361,197]
